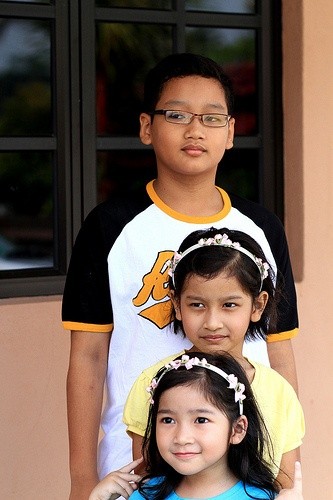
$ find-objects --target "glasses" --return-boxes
[147,109,231,127]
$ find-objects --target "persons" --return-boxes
[60,53,300,500]
[88,350,295,500]
[122,226,308,499]
